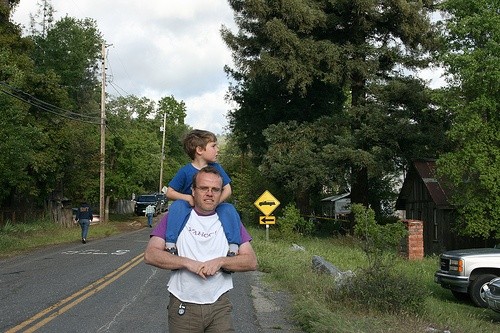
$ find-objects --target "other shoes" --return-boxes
[223,251,239,274]
[165,248,178,256]
[82,239,86,245]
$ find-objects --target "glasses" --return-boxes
[194,185,222,194]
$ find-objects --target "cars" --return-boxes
[73,208,99,226]
[487,279,500,314]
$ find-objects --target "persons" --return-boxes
[144,167,257,332]
[163,129,238,256]
[146,202,155,227]
[76,203,93,243]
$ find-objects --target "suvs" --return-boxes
[155,193,169,212]
[136,193,158,214]
[435,248,500,306]
